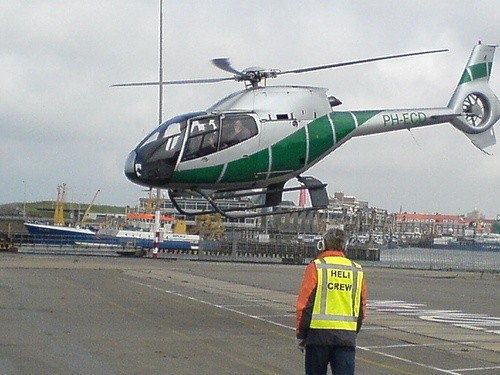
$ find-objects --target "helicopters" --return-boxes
[111,41,499,219]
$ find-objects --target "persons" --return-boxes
[295,229,368,375]
[233,120,251,142]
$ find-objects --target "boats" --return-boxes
[23,217,200,250]
